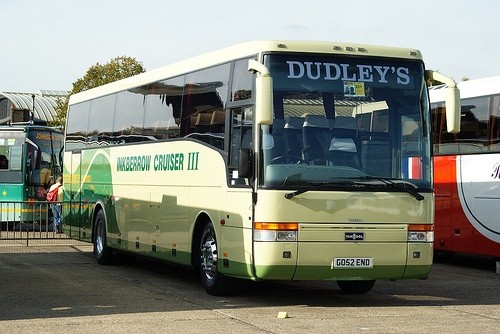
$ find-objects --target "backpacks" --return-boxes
[46,184,62,204]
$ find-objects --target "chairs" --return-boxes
[120,111,375,165]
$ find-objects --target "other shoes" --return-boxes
[54,223,63,234]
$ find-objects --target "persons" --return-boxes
[48,177,63,233]
